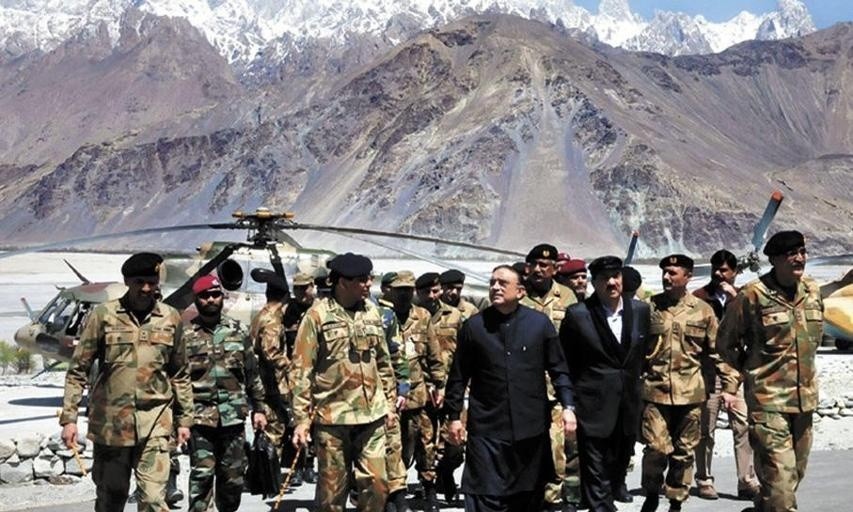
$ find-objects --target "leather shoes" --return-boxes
[698,486,717,499]
[614,487,632,502]
[739,486,761,500]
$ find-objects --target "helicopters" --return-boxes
[0,190,852,510]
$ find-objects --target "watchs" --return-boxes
[561,406,576,413]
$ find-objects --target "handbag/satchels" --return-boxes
[256,432,282,498]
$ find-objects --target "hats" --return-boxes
[660,255,693,267]
[764,231,804,255]
[192,276,220,295]
[267,253,373,290]
[512,243,586,276]
[121,252,163,277]
[589,256,640,291]
[382,269,464,289]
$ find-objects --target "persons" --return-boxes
[446,267,576,511]
[636,253,719,511]
[176,275,267,512]
[58,250,193,512]
[247,241,479,511]
[688,249,763,501]
[715,228,829,511]
[482,244,589,510]
[559,254,653,512]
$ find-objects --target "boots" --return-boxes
[166,471,183,504]
[290,461,304,485]
[394,484,439,512]
